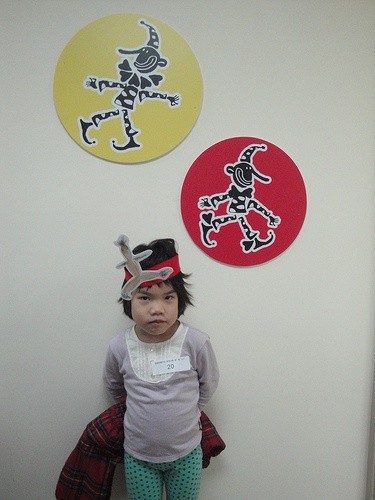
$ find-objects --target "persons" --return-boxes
[101,237,221,500]
